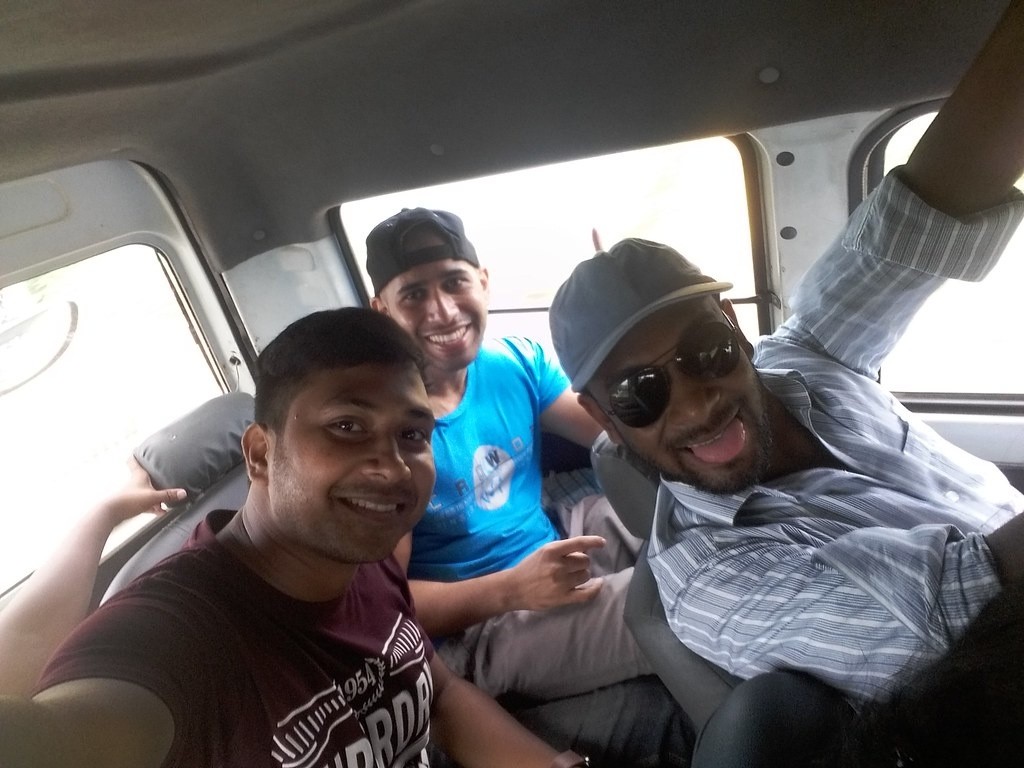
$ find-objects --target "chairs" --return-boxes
[99,391,259,613]
[590,430,740,731]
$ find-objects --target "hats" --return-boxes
[366,206,479,293]
[548,236,734,393]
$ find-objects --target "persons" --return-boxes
[548,0,1024,710]
[0,306,588,768]
[365,209,654,700]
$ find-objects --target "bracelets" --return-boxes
[549,745,588,768]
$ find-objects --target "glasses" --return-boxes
[608,321,741,429]
